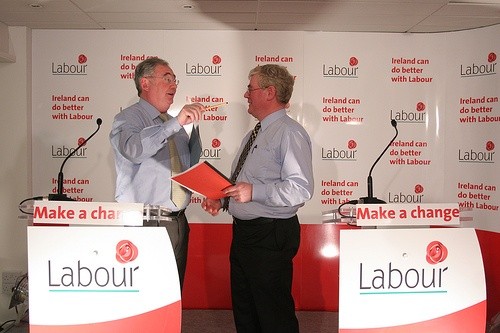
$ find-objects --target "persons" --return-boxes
[201,64,314,333]
[108,57,206,299]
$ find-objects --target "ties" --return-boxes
[157,113,187,210]
[223,122,261,212]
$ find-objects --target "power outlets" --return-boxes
[1,272,28,295]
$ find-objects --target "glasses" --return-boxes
[246,87,274,94]
[141,76,180,86]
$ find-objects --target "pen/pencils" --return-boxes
[206,101,228,110]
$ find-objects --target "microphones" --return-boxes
[48,118,102,201]
[358,120,398,204]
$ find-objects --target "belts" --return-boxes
[231,215,301,226]
[142,208,187,215]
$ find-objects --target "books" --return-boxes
[170,161,234,200]
